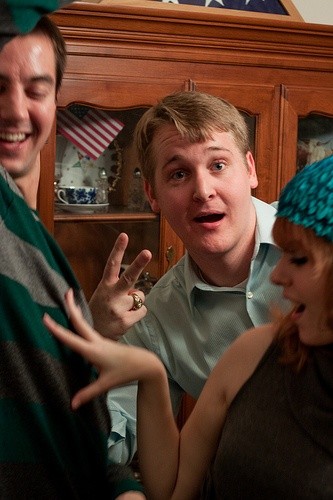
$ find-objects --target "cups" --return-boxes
[57,185,99,204]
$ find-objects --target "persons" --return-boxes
[0,0,143,500]
[0,15,151,343]
[43,155,333,500]
[100,93,302,469]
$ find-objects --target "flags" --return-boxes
[56,104,125,161]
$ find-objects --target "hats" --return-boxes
[274,154,333,239]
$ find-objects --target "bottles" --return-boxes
[96,167,108,204]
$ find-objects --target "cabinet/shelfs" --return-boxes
[39,0,333,413]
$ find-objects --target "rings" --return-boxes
[132,294,142,310]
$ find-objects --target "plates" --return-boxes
[55,203,110,213]
